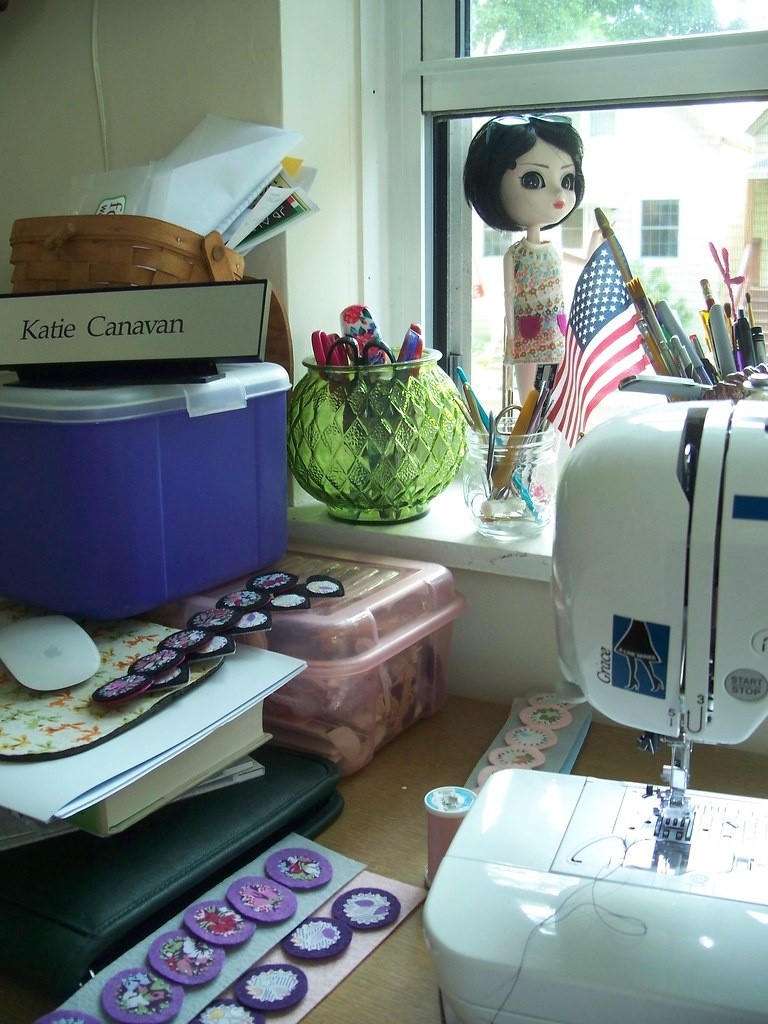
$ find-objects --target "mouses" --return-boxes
[0,614,102,691]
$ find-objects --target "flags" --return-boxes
[540,236,666,448]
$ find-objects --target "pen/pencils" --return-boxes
[595,207,767,401]
[397,324,424,382]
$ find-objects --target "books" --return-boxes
[0,621,309,853]
[215,164,283,236]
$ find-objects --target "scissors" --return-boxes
[311,329,349,393]
[325,339,398,434]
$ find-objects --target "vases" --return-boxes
[284,346,468,524]
[465,418,570,545]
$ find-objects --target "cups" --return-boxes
[465,417,571,540]
[424,785,476,883]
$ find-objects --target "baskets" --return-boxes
[11,215,245,297]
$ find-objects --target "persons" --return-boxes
[461,111,586,412]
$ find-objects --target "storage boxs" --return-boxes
[0,278,271,383]
[0,361,294,621]
[152,539,467,778]
